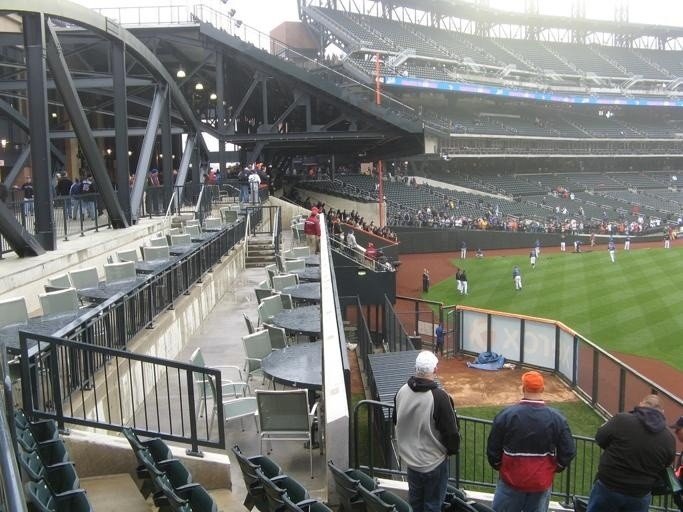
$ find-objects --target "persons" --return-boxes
[457,269,467,294]
[549,184,573,198]
[399,199,683,269]
[435,322,445,357]
[14,176,33,216]
[380,158,416,187]
[512,268,522,292]
[585,396,677,511]
[392,351,459,512]
[487,371,576,511]
[55,170,221,222]
[669,415,683,509]
[238,162,276,206]
[284,164,327,183]
[305,198,398,271]
[423,268,430,294]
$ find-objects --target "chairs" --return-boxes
[407,105,683,140]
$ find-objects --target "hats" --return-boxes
[522,371,545,393]
[415,351,438,373]
[670,417,683,429]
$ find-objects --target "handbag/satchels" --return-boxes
[652,466,683,496]
